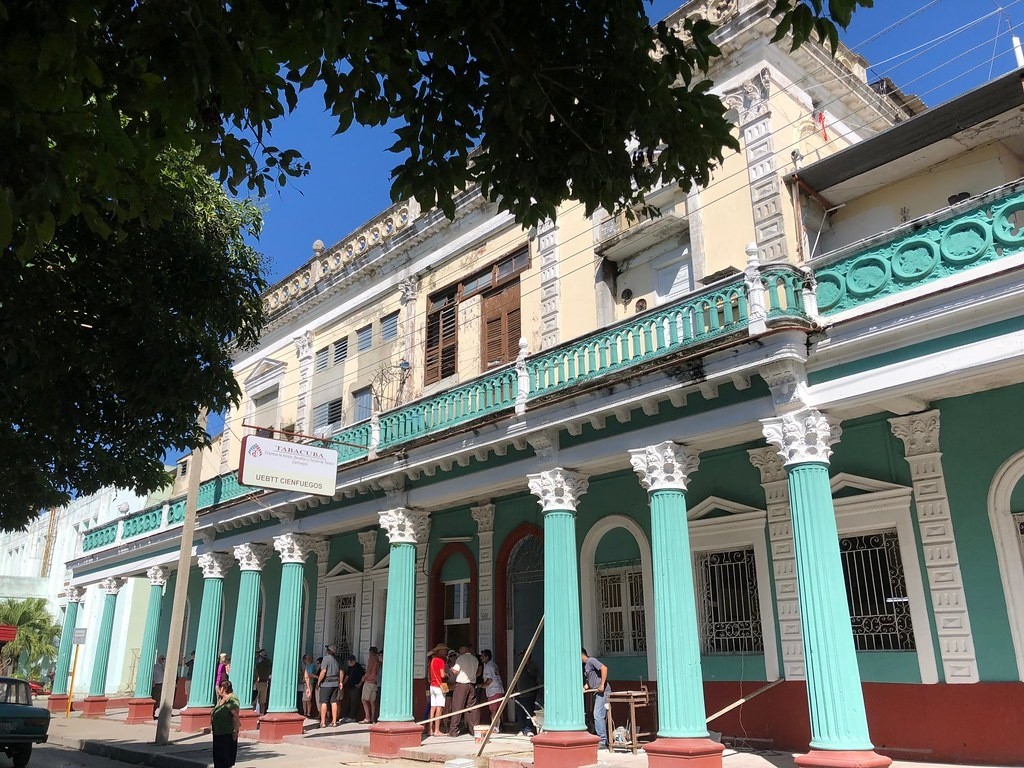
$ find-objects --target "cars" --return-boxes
[0,676,51,768]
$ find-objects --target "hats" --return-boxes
[446,649,459,660]
[427,648,436,657]
[325,644,336,653]
[481,650,492,658]
[458,641,470,648]
[520,649,532,658]
[433,643,451,650]
[260,651,268,658]
[346,655,355,660]
[377,650,383,654]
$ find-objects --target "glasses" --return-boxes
[317,663,320,665]
[448,654,456,658]
[162,658,165,660]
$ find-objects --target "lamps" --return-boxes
[439,537,474,543]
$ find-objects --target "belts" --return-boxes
[456,682,475,685]
[155,683,162,685]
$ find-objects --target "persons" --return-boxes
[513,648,538,737]
[152,655,166,719]
[298,643,383,729]
[210,680,240,768]
[581,647,611,749]
[252,649,273,716]
[179,650,195,710]
[421,643,506,737]
[214,652,231,705]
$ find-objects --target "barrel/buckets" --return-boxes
[473,724,490,744]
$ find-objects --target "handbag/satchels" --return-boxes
[425,690,430,697]
[441,683,449,693]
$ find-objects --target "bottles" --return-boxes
[618,730,622,743]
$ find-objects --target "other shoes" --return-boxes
[422,723,473,737]
[527,731,534,737]
[493,727,505,733]
[517,732,524,736]
[303,713,376,728]
